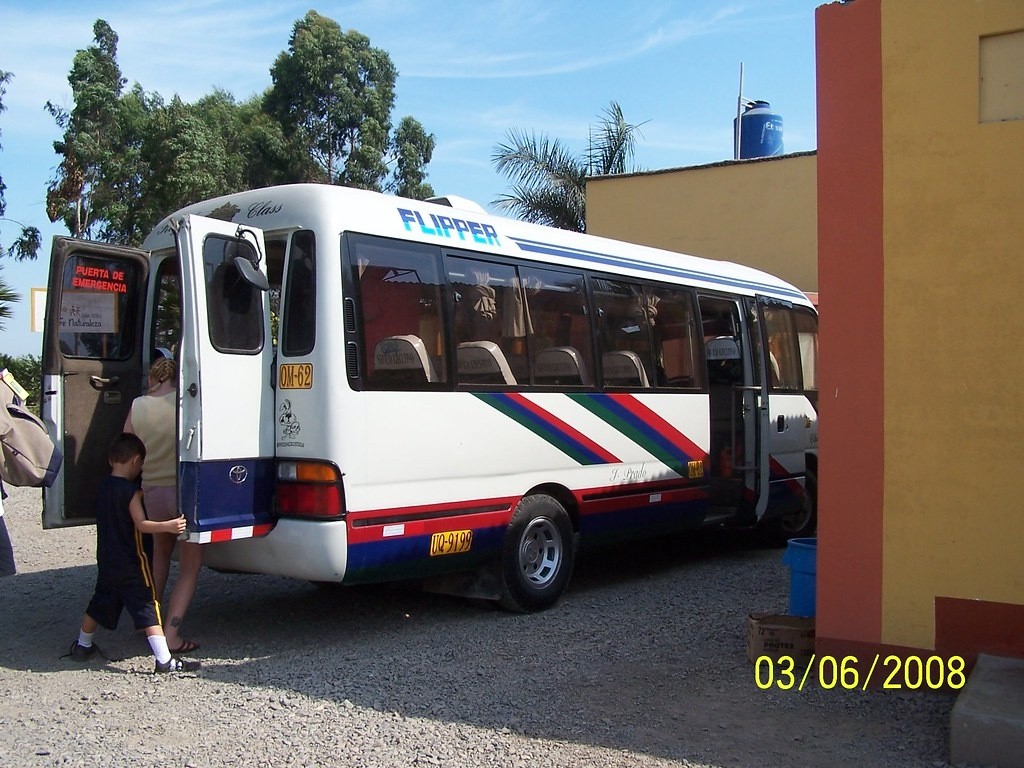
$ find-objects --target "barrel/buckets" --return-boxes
[733,101,784,159]
[783,537,817,616]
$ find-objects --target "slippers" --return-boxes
[170,639,199,654]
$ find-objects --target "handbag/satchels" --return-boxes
[0,374,63,488]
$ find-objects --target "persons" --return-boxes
[69,432,202,672]
[120,357,201,653]
[0,363,19,578]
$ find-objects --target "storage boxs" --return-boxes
[746,612,815,667]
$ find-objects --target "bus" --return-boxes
[42,183,820,614]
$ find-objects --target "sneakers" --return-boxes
[59,639,124,662]
[155,654,201,672]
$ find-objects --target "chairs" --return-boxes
[700,336,781,431]
[532,347,602,386]
[601,351,651,386]
[371,334,439,384]
[452,339,520,385]
